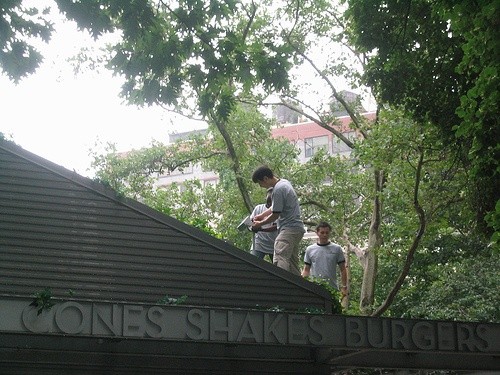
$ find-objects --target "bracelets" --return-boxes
[342,286,347,290]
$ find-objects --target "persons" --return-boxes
[252,165,306,276]
[301,221,349,296]
[250,187,279,264]
